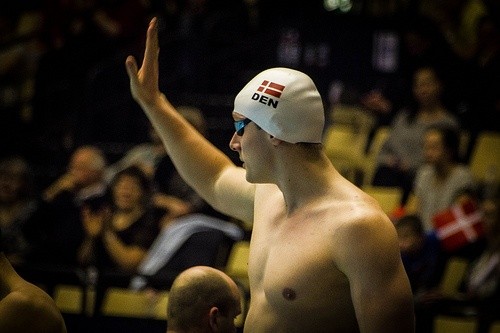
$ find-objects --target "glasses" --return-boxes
[234,117,251,137]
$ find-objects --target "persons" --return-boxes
[0,291,65,333]
[125,16,418,333]
[76,165,158,323]
[168,266,243,333]
[0,66,500,333]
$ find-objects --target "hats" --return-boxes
[234,67,325,144]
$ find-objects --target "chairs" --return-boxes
[55,124,500,333]
